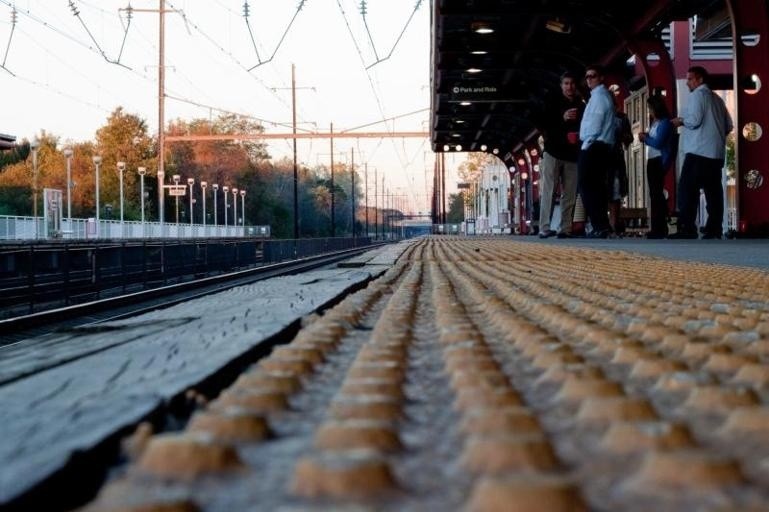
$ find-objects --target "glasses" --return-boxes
[585,73,597,78]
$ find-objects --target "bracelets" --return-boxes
[677,118,685,126]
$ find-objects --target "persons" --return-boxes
[669,65,733,241]
[529,62,634,239]
[636,94,674,239]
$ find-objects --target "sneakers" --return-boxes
[539,226,613,239]
[646,231,722,239]
